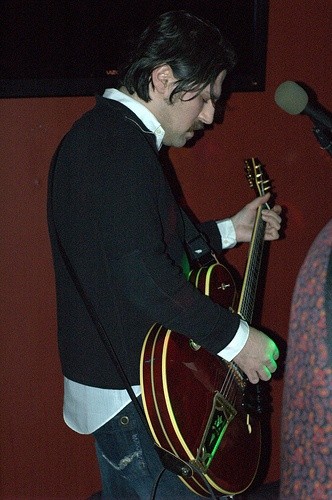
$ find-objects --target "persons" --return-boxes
[44,7,284,500]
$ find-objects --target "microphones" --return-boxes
[276,81,332,144]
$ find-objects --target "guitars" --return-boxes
[139,157,275,500]
